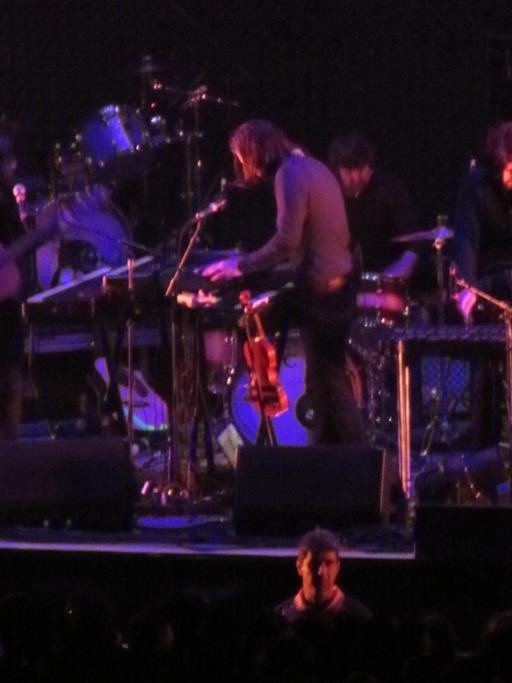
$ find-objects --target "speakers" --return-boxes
[232,444,387,538]
[0,437,137,534]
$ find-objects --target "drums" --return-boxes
[224,328,381,446]
[81,101,154,166]
[355,273,410,331]
[28,190,134,290]
[13,169,48,221]
[46,133,93,188]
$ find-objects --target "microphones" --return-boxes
[193,196,227,221]
[12,183,30,231]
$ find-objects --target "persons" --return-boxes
[2,527,511,683]
[326,129,423,268]
[2,162,151,315]
[190,116,377,447]
[447,117,512,325]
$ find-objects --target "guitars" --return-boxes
[95,357,171,441]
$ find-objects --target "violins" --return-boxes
[239,289,289,418]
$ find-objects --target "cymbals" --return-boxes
[392,227,453,242]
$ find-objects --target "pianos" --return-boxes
[22,247,300,326]
